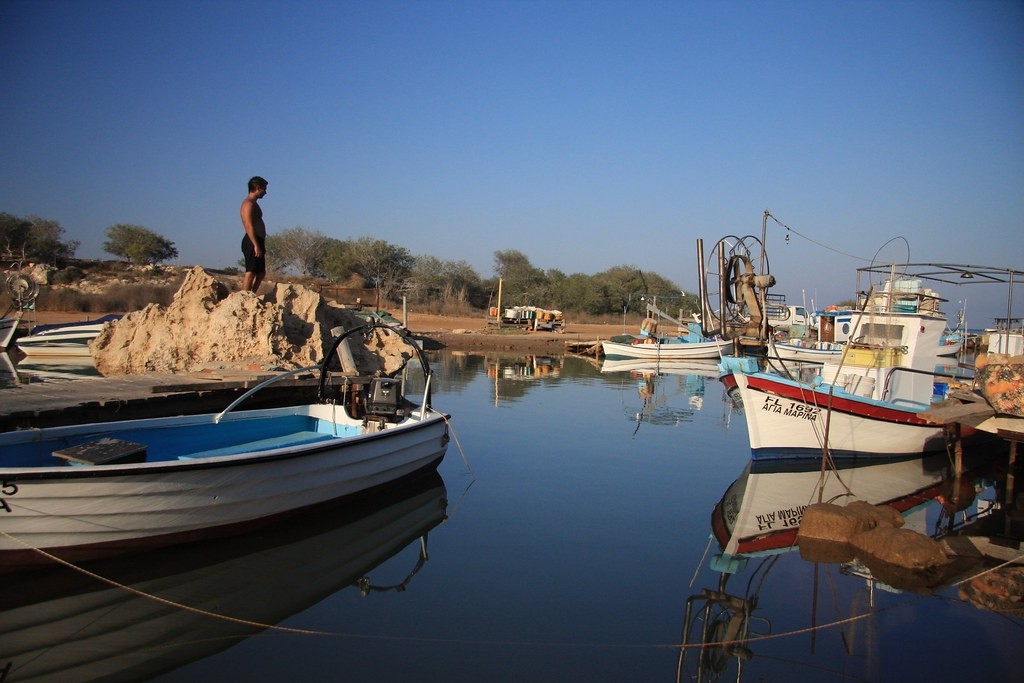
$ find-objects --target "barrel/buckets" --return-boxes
[845,374,875,399]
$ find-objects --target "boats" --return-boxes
[693,207,1024,463]
[599,358,721,441]
[674,452,1002,683]
[0,296,452,570]
[0,469,453,683]
[601,286,964,362]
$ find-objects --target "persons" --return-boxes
[240,176,268,293]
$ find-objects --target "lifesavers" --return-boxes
[825,305,839,312]
